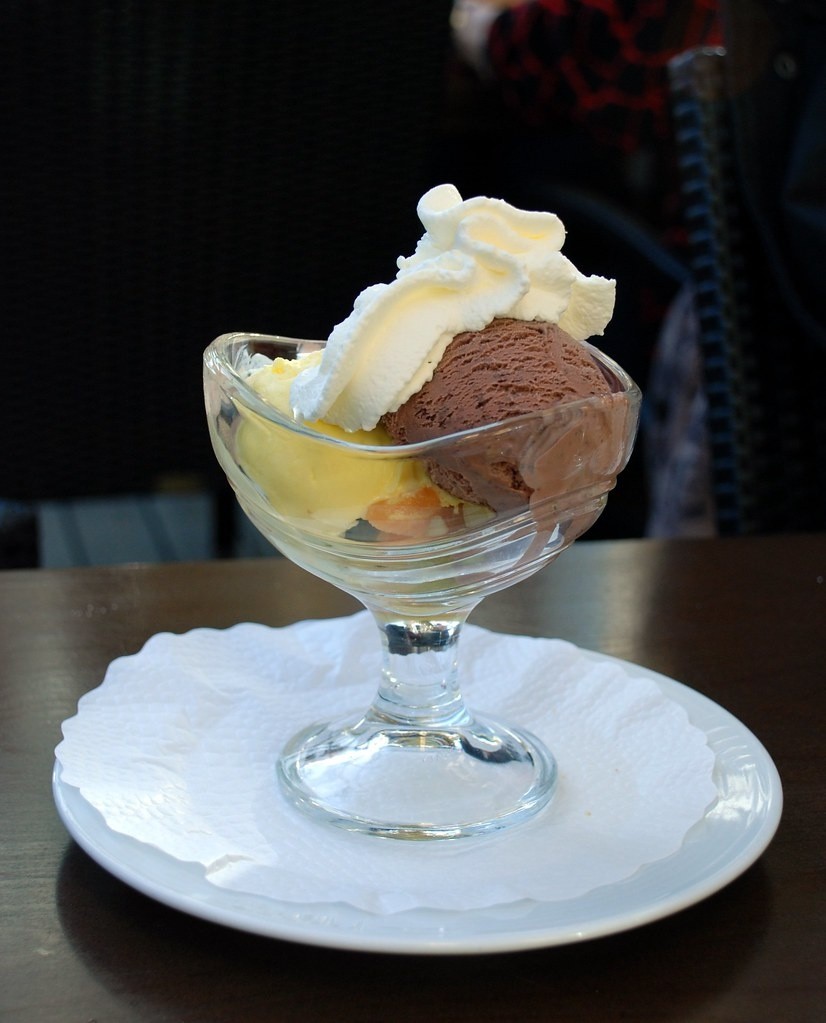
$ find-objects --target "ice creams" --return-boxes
[233,182,630,550]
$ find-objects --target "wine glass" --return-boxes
[204,331,641,840]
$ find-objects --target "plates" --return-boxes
[54,634,783,955]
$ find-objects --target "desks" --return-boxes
[0,534,826,1023]
